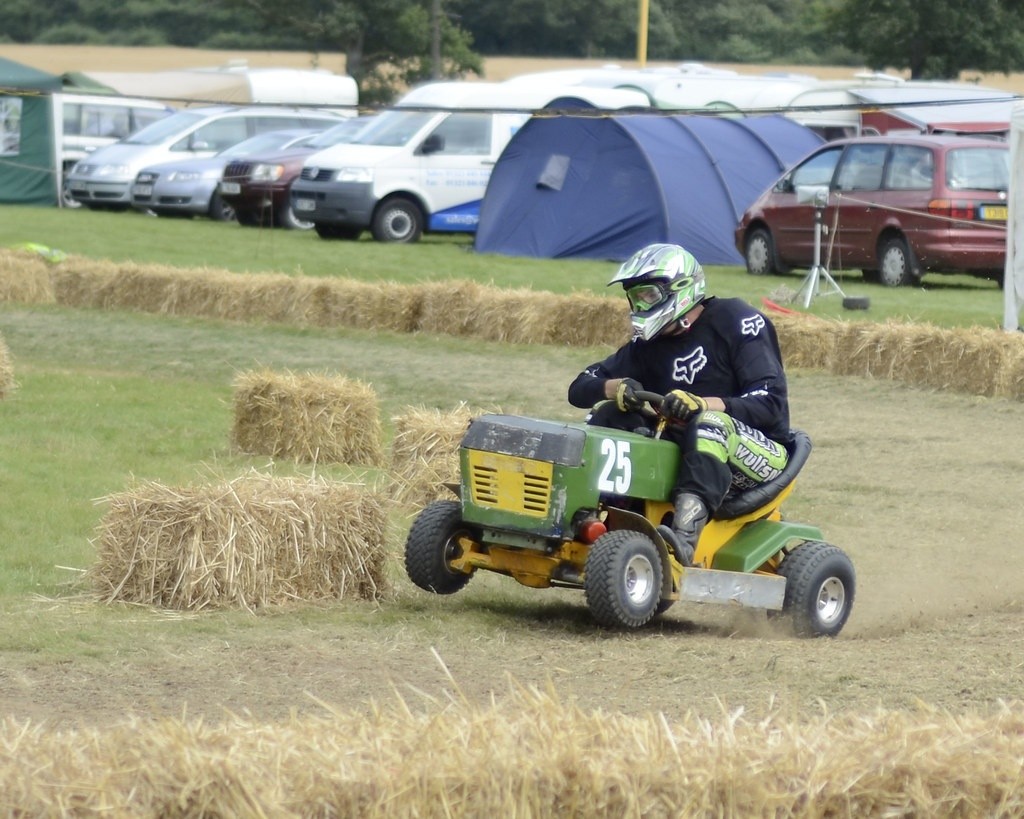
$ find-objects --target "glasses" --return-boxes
[626,281,667,312]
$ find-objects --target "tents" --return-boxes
[475,96,839,265]
[0,57,358,208]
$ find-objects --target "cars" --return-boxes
[221,117,375,230]
[132,128,322,225]
[733,135,1013,290]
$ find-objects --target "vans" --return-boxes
[68,106,340,212]
[289,81,654,248]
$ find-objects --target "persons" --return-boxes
[568,244,791,568]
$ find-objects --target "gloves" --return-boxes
[661,390,708,422]
[615,377,658,419]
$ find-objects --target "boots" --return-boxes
[656,490,709,567]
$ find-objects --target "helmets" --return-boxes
[609,243,706,340]
